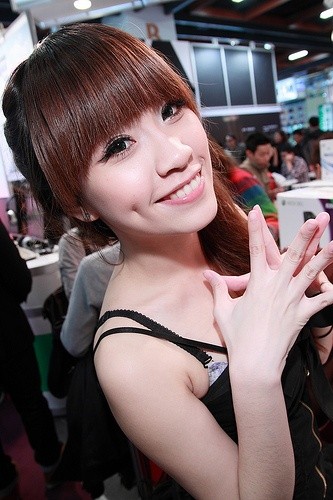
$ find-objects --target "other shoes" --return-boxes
[46,481,64,491]
[0,474,19,498]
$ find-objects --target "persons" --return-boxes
[2,23,333,500]
[13,116,332,355]
[1,221,59,500]
